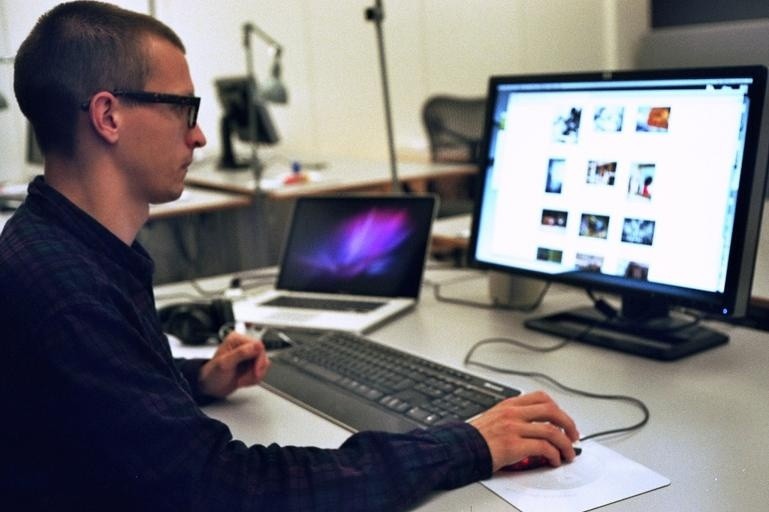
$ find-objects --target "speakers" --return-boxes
[489,264,543,309]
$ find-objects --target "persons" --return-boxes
[0,0,580,512]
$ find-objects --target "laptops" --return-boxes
[231,194,441,337]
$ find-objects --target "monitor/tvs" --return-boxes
[465,64,769,361]
[214,77,278,168]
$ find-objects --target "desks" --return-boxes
[0,164,478,282]
[0,203,769,511]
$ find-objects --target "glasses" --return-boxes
[81,89,201,127]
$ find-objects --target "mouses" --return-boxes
[498,421,582,472]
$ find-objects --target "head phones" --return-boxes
[157,298,235,346]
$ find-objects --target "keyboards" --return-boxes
[256,329,525,434]
